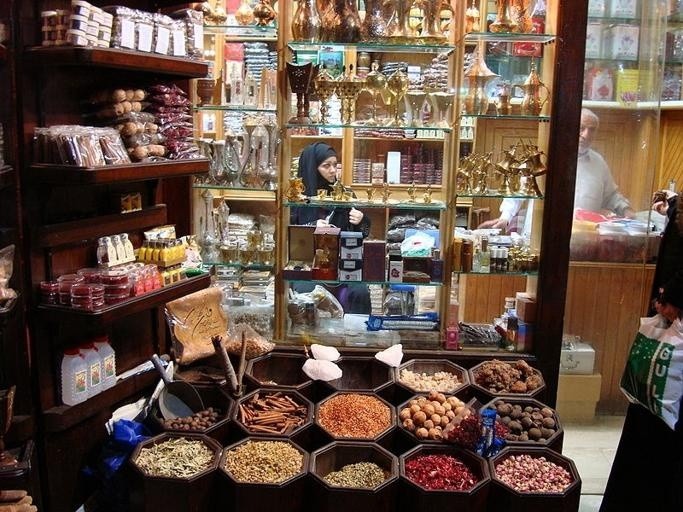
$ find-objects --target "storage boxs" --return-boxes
[557,340,596,376]
[554,371,602,426]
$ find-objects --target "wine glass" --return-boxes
[285,54,451,131]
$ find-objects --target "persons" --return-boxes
[290,142,371,242]
[477,108,637,243]
[597,190,683,512]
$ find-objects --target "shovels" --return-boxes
[151,353,205,421]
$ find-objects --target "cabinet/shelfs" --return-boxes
[579,12,682,110]
[179,1,590,362]
[0,0,212,511]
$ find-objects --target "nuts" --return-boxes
[401,390,471,440]
[164,407,219,432]
[489,401,556,444]
[89,88,166,159]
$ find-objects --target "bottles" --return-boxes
[59,335,118,407]
[95,233,133,263]
[473,239,490,272]
[505,317,518,351]
